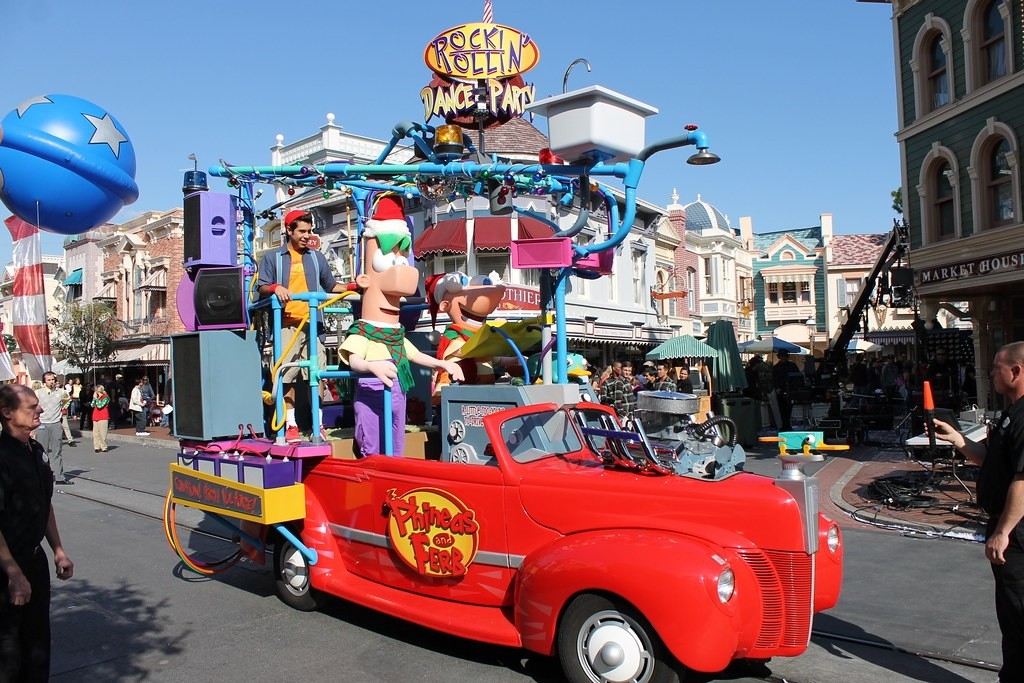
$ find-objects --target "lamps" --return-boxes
[737,298,752,303]
[687,266,696,273]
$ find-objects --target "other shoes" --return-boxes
[101,449,107,452]
[95,449,101,452]
[135,431,151,436]
[68,441,77,447]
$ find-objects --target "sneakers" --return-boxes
[284,424,302,442]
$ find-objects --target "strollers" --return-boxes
[143,397,165,428]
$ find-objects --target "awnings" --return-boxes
[92,281,118,303]
[412,216,556,262]
[133,269,166,292]
[61,268,83,286]
[94,343,171,368]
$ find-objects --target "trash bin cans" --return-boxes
[722,397,756,448]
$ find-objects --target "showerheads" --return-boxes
[683,122,722,165]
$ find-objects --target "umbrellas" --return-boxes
[844,337,882,353]
[737,331,811,356]
[644,334,720,365]
[50,355,92,382]
[706,319,750,398]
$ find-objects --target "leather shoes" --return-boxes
[55,479,74,485]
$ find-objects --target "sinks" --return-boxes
[523,84,659,166]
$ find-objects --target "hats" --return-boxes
[357,193,415,254]
[285,210,309,229]
[887,354,894,359]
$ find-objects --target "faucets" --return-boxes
[562,57,592,93]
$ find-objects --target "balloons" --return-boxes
[0,92,140,235]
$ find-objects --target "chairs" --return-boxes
[119,398,134,424]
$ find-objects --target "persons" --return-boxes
[55,374,174,436]
[56,380,78,447]
[91,384,110,454]
[32,371,76,485]
[924,340,1024,683]
[424,271,529,406]
[585,359,694,442]
[338,198,465,457]
[254,208,363,442]
[745,349,978,432]
[0,383,73,683]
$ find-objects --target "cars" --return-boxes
[168,395,851,683]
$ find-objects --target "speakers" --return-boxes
[170,329,266,440]
[177,190,248,330]
[573,245,614,275]
[511,238,573,268]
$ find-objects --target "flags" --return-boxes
[0,319,19,383]
[4,214,53,380]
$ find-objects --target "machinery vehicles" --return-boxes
[763,217,909,442]
